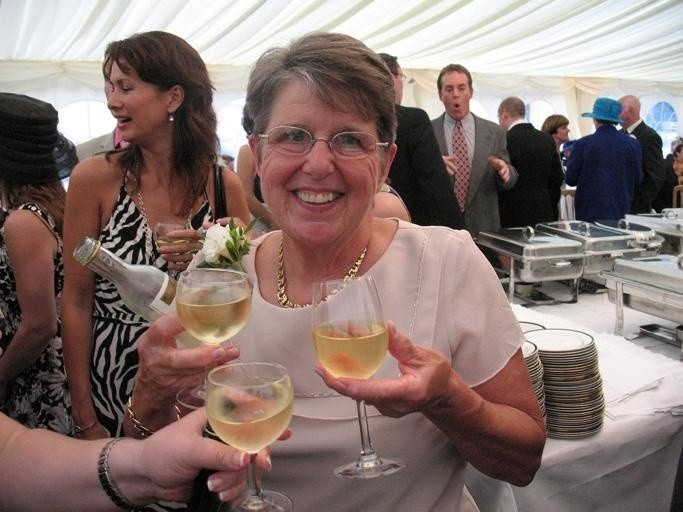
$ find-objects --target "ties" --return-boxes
[452,122,471,214]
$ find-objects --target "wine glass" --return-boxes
[174,267,254,410]
[205,362,296,512]
[311,274,407,481]
[154,221,191,278]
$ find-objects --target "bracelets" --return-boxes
[97,437,147,511]
[127,394,182,436]
[74,421,97,433]
[58,32,250,438]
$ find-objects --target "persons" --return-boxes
[497,93,564,227]
[377,51,464,230]
[121,30,546,512]
[0,384,292,512]
[372,181,412,221]
[235,143,279,239]
[429,62,519,233]
[542,94,683,221]
[0,90,82,437]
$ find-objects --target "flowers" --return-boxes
[184,216,263,290]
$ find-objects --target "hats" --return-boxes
[582,99,624,123]
[1,92,78,184]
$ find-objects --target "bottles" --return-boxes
[73,236,204,348]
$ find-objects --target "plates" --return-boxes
[517,321,604,438]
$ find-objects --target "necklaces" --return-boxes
[135,190,193,267]
[275,237,366,309]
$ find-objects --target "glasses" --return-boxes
[258,126,389,161]
